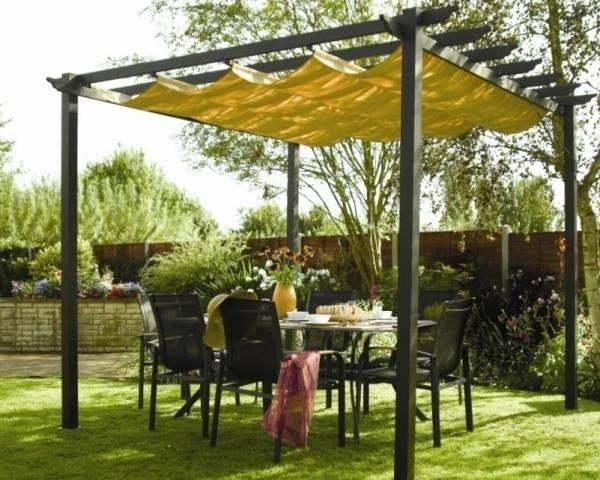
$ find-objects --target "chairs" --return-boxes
[136,290,239,438]
[305,290,356,368]
[361,291,464,422]
[210,291,345,465]
[356,296,474,447]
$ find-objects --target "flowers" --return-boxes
[256,246,314,288]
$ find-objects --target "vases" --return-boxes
[271,280,297,320]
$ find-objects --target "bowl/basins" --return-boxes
[309,314,330,324]
[372,311,392,319]
[287,311,308,320]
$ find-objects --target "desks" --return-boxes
[252,314,439,444]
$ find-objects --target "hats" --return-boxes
[202,290,258,348]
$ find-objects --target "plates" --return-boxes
[306,322,337,327]
[369,320,398,324]
[286,319,309,323]
